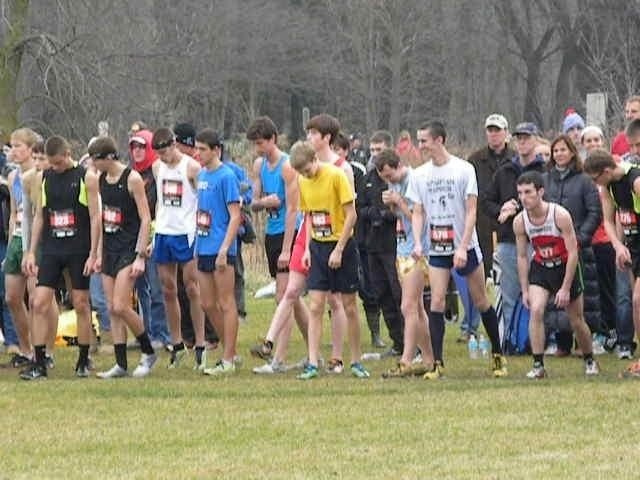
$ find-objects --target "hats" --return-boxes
[563,108,585,134]
[485,114,508,129]
[512,122,536,136]
[128,136,146,145]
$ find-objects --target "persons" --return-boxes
[245,95,639,381]
[4,121,255,380]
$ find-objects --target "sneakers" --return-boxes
[0,339,242,380]
[456,331,640,379]
[250,340,445,379]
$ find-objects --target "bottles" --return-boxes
[469,335,478,360]
[478,334,489,360]
